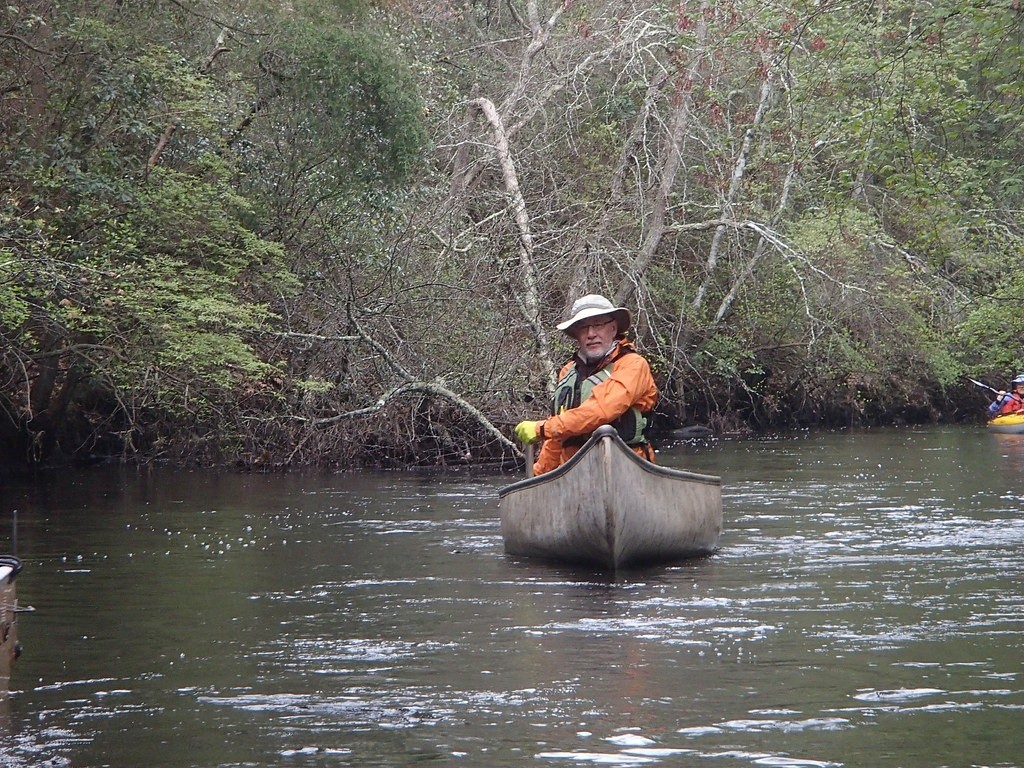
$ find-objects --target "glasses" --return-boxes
[573,318,615,331]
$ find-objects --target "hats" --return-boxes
[1010,375,1024,383]
[555,294,631,340]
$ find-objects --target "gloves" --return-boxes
[515,420,537,445]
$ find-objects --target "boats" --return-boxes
[987,414,1024,434]
[498,424,723,570]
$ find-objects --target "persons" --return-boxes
[989,375,1024,415]
[515,294,658,477]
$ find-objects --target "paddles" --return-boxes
[958,374,1024,405]
